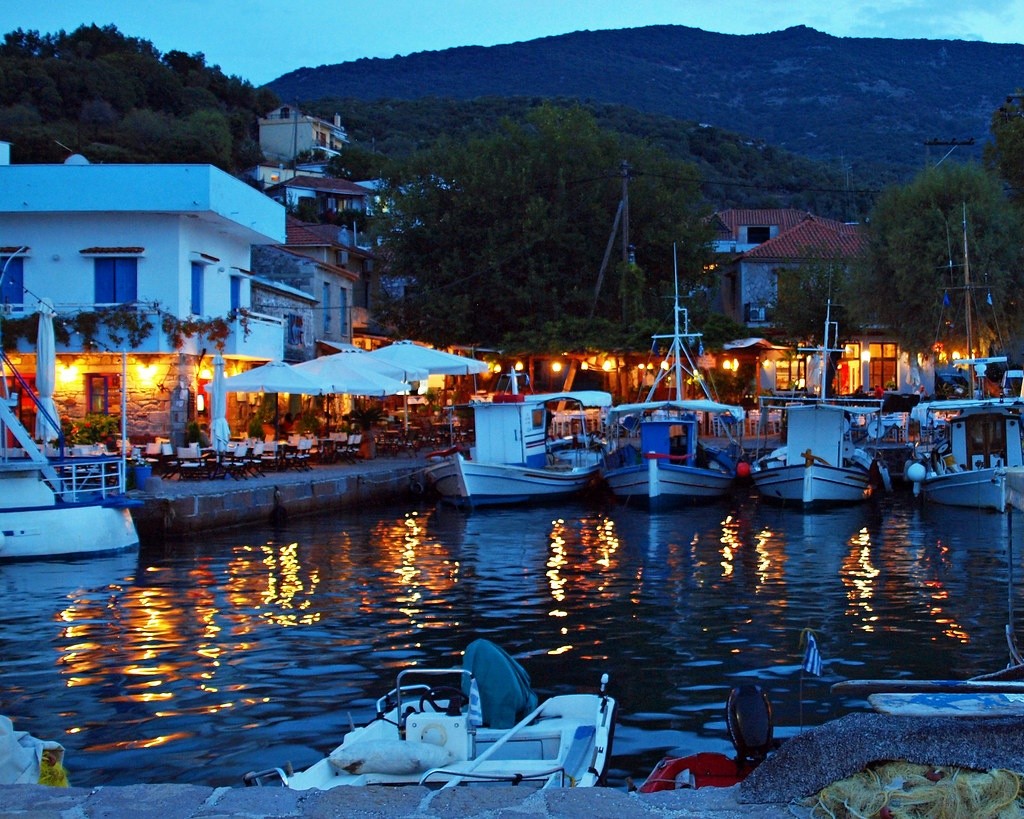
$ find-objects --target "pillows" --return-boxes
[328,740,463,773]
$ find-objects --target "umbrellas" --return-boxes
[202,359,321,442]
[34,298,61,457]
[370,337,488,428]
[317,345,429,431]
[209,350,231,464]
[290,354,386,439]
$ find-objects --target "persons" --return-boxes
[184,404,426,450]
[760,383,984,401]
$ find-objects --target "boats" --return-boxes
[236,637,620,792]
[0,265,145,561]
[426,197,1023,510]
[625,635,1024,794]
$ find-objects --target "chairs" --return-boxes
[727,683,784,774]
[1,384,964,481]
